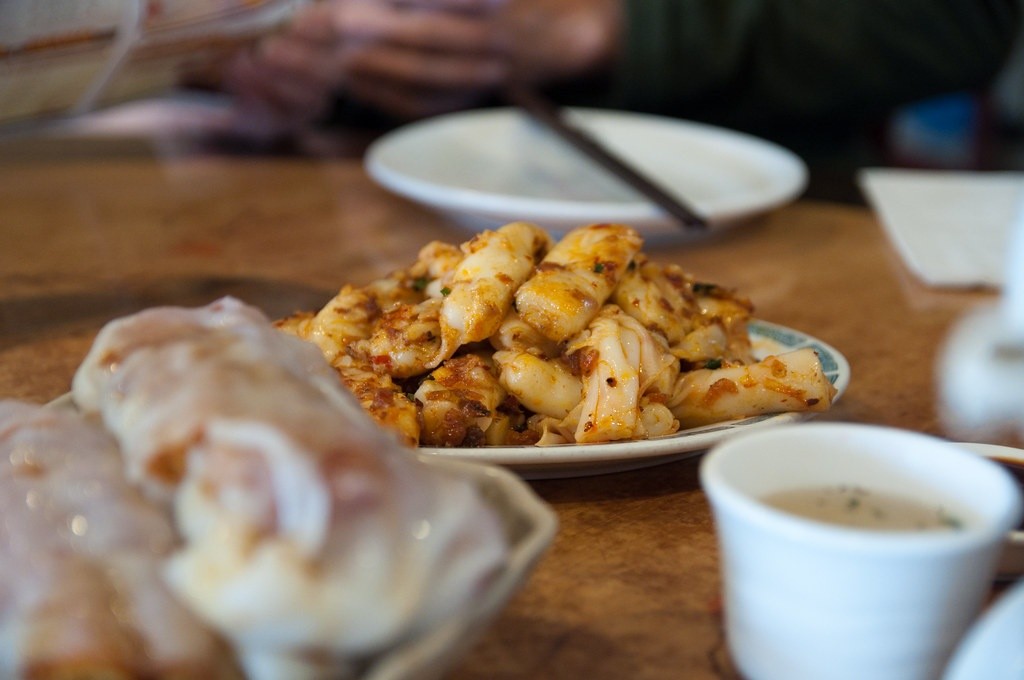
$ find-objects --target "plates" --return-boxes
[955,440,1024,542]
[862,171,1024,287]
[420,315,851,478]
[363,455,559,680]
[364,108,808,248]
[943,581,1024,680]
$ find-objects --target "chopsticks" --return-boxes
[526,104,709,229]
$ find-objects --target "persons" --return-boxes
[128,0,1024,198]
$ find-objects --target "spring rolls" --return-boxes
[0,292,512,680]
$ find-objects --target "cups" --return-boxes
[702,422,1024,680]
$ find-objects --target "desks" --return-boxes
[0,156,998,680]
